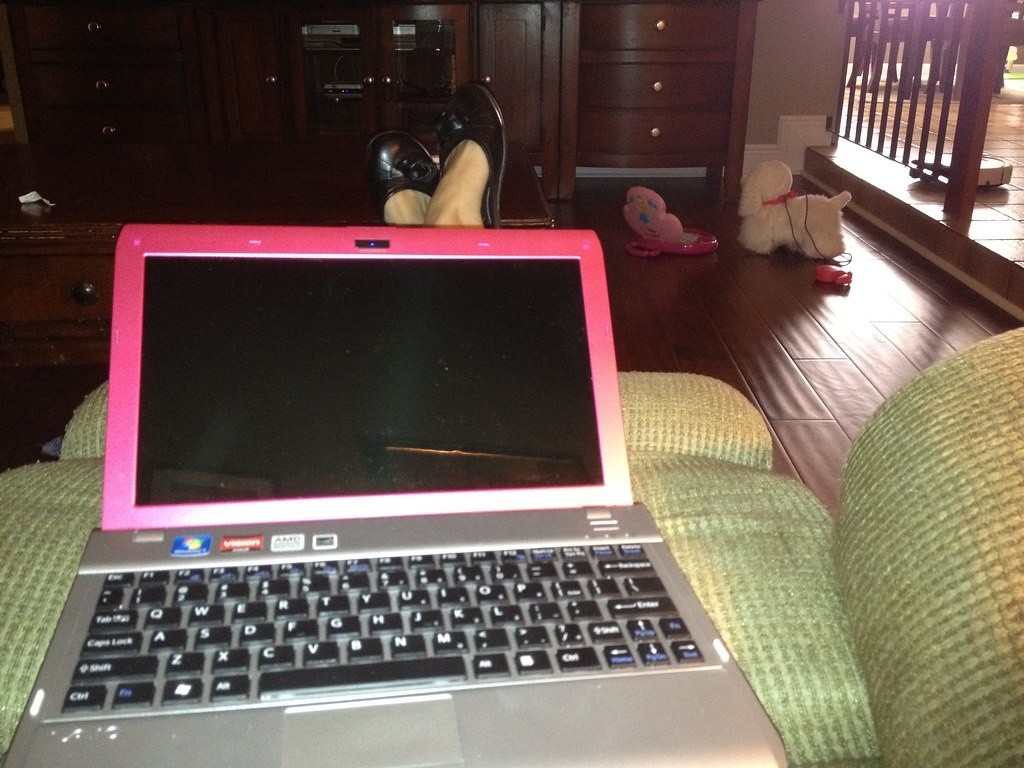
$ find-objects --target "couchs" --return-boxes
[1,325,1022,768]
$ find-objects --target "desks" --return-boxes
[1,151,556,399]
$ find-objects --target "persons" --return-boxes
[365,84,507,226]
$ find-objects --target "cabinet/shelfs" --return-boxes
[199,2,558,199]
[558,1,758,203]
[1,0,205,148]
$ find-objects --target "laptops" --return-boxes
[1,223,786,768]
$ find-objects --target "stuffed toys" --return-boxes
[736,161,852,262]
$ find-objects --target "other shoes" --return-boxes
[434,81,504,229]
[368,132,440,225]
